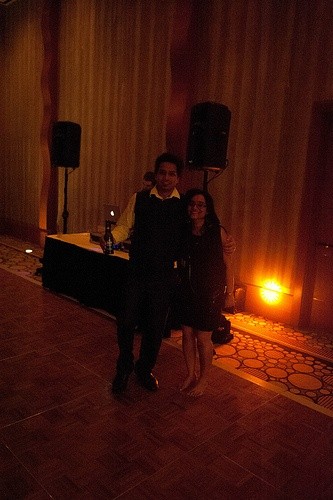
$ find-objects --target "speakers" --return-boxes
[51,121,80,169]
[186,102,231,172]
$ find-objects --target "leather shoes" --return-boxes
[112,358,134,394]
[134,360,159,391]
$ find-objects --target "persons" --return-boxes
[100,152,236,396]
[178,187,236,397]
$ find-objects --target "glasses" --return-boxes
[188,202,207,209]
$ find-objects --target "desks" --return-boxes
[40,232,185,340]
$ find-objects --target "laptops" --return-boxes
[102,204,122,223]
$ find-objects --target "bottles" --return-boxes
[104,225,113,254]
